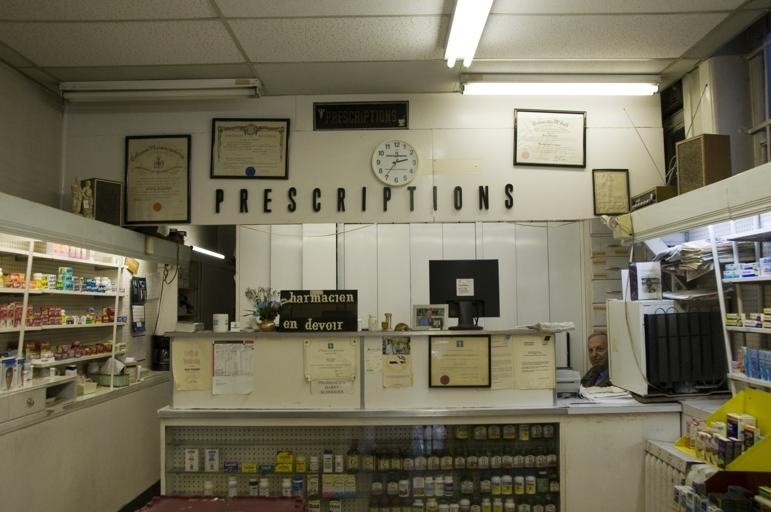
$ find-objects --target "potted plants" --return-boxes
[241,286,281,331]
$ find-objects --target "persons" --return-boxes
[418,310,433,327]
[579,331,609,387]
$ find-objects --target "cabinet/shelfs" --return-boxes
[632,157,771,397]
[0,190,201,512]
[745,40,771,166]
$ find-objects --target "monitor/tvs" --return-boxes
[429,258,501,330]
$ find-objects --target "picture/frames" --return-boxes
[412,303,449,331]
[513,106,587,169]
[427,335,492,389]
[591,168,631,216]
[123,134,192,224]
[210,117,292,179]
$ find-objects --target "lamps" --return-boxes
[456,73,663,98]
[58,77,265,102]
[442,0,496,69]
[190,245,225,260]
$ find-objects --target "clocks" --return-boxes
[372,138,420,186]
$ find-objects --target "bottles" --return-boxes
[259,478,270,497]
[227,477,238,497]
[358,315,362,332]
[367,314,378,332]
[281,445,560,511]
[248,479,258,497]
[203,480,214,497]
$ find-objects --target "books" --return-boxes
[512,320,574,334]
[578,384,635,406]
[651,235,755,301]
